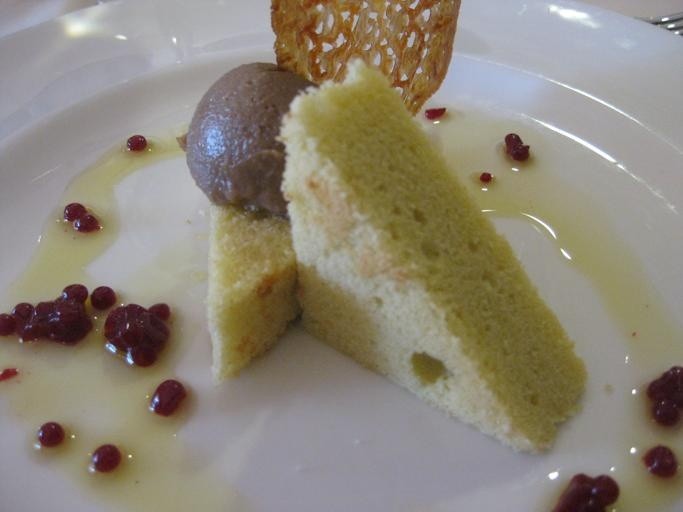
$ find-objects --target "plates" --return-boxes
[1,2,681,510]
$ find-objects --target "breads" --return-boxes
[207,203,300,383]
[276,58,587,456]
